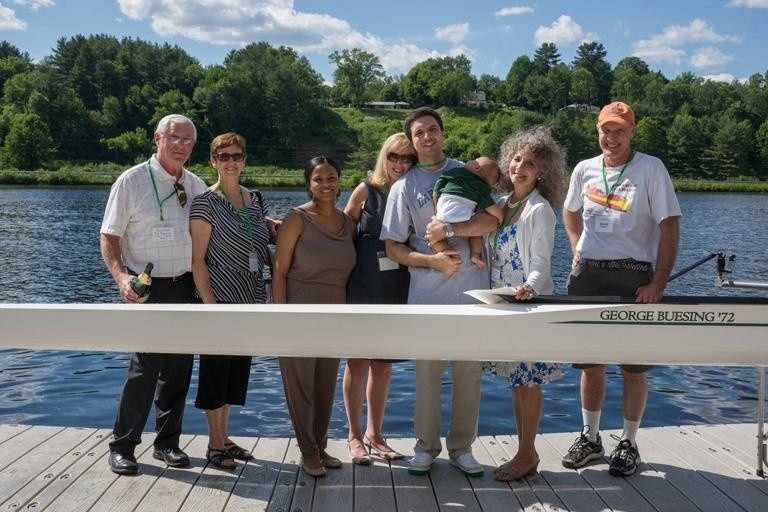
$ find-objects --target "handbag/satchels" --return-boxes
[360,211,376,238]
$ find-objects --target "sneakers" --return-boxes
[408,452,433,475]
[562,438,604,468]
[609,440,639,476]
[449,452,485,477]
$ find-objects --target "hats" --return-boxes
[598,102,635,128]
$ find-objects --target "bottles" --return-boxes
[130,262,153,303]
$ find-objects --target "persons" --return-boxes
[378,104,499,477]
[342,132,418,466]
[188,131,273,468]
[270,154,357,477]
[562,101,682,475]
[483,124,566,482]
[99,114,283,475]
[431,155,501,272]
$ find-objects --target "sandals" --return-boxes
[225,442,254,460]
[206,446,237,469]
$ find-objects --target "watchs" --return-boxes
[443,222,456,237]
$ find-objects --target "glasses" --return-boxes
[216,153,246,161]
[387,152,417,163]
[174,182,187,207]
[168,136,194,145]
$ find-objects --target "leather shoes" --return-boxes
[302,449,341,476]
[154,447,190,467]
[109,452,138,474]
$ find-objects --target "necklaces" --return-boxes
[507,195,527,208]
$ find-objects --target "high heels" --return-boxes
[348,439,372,465]
[494,450,538,482]
[363,434,405,459]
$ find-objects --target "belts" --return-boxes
[127,269,189,283]
[580,258,650,272]
